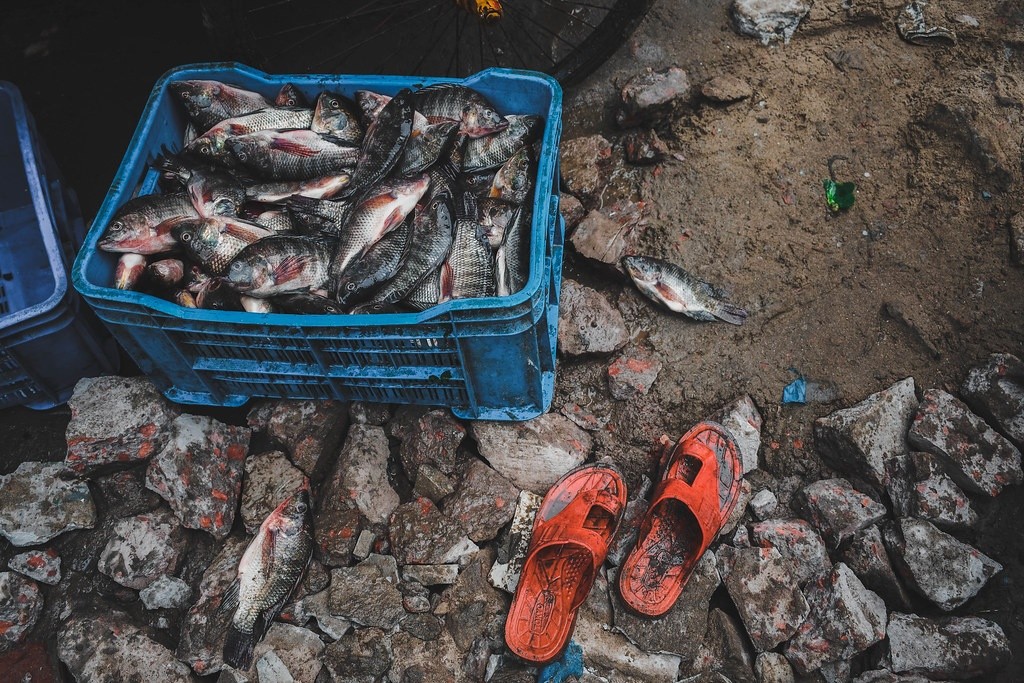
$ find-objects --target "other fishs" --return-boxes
[96,81,545,315]
[620,254,747,326]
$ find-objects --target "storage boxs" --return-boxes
[0,78,122,416]
[68,61,566,421]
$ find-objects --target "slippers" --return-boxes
[504,462,627,667]
[614,420,743,621]
